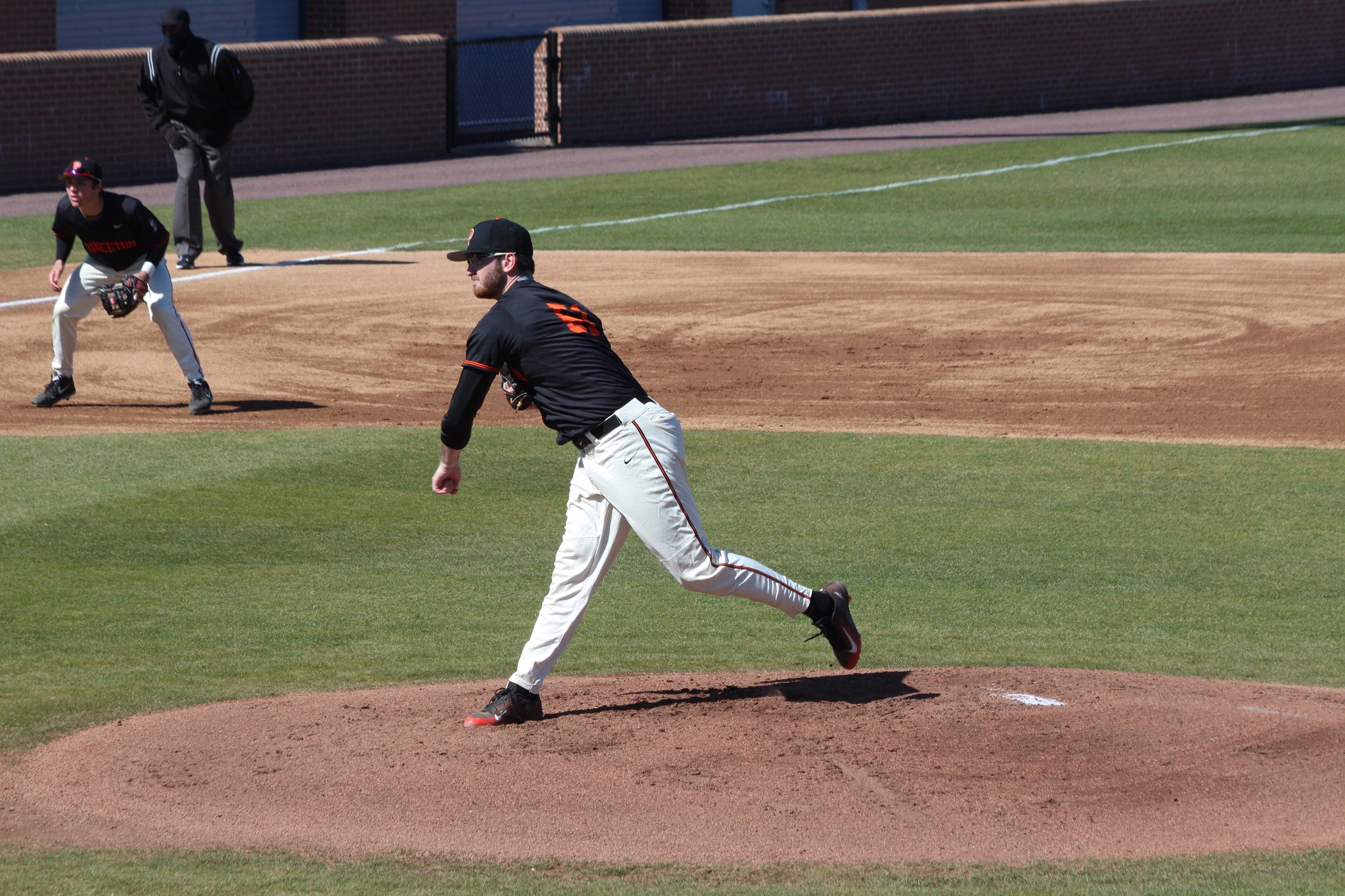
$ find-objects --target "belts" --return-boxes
[572,396,659,450]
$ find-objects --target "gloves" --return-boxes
[158,121,188,149]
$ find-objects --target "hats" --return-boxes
[160,8,190,27]
[58,157,103,182]
[447,217,533,262]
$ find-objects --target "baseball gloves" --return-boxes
[98,275,148,318]
[499,361,531,410]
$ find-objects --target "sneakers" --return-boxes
[188,380,212,415]
[225,252,244,266]
[463,681,543,727]
[30,374,76,407]
[810,580,862,670]
[176,255,195,269]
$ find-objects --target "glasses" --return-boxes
[467,252,516,266]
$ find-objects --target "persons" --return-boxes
[35,156,213,415]
[144,8,257,271]
[429,216,862,728]
